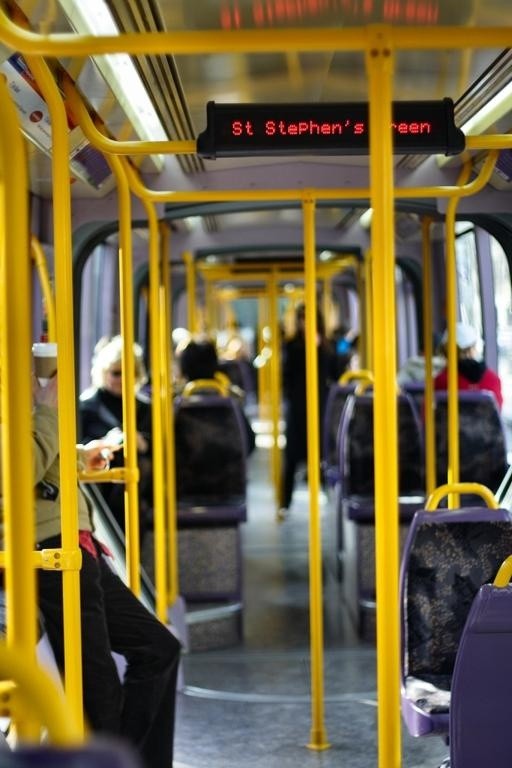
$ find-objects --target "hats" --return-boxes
[442,322,479,349]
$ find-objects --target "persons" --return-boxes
[172,344,254,457]
[420,323,504,425]
[79,335,151,554]
[278,301,334,519]
[0,352,181,768]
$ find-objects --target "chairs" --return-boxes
[322,365,511,527]
[449,554,512,767]
[399,481,512,747]
[140,379,248,528]
